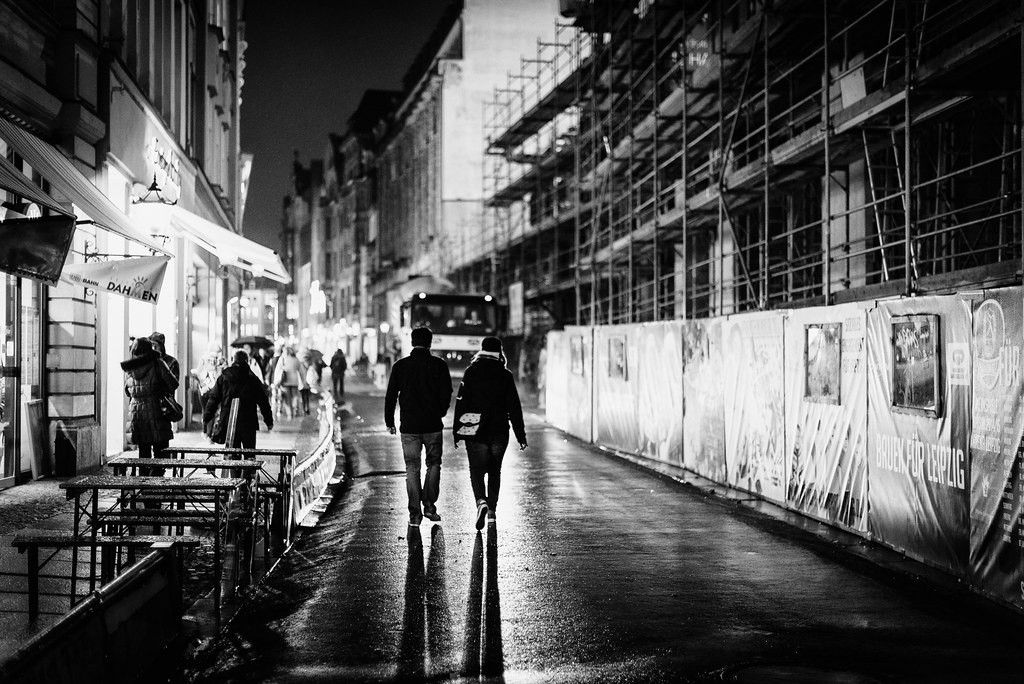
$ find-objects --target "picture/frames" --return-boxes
[890,314,940,421]
[803,323,844,406]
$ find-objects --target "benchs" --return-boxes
[12,481,292,643]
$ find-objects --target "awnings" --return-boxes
[135,201,291,283]
[0,117,173,257]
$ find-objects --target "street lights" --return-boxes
[380,322,388,367]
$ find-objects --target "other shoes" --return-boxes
[424,510,440,521]
[476,504,489,529]
[410,520,419,527]
[488,514,496,522]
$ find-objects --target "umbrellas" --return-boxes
[231,336,275,349]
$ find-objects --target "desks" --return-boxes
[58,474,245,611]
[106,457,268,527]
[160,445,299,523]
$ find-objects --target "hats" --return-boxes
[148,331,165,344]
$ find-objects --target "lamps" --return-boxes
[132,172,179,249]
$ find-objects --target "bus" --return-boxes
[399,294,503,396]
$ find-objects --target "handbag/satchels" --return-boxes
[160,391,183,422]
[211,403,228,444]
[457,412,483,439]
[281,357,287,383]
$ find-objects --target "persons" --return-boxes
[197,344,271,494]
[149,332,180,380]
[385,327,453,526]
[361,354,368,361]
[267,344,328,420]
[331,349,347,398]
[125,338,179,509]
[453,337,528,530]
